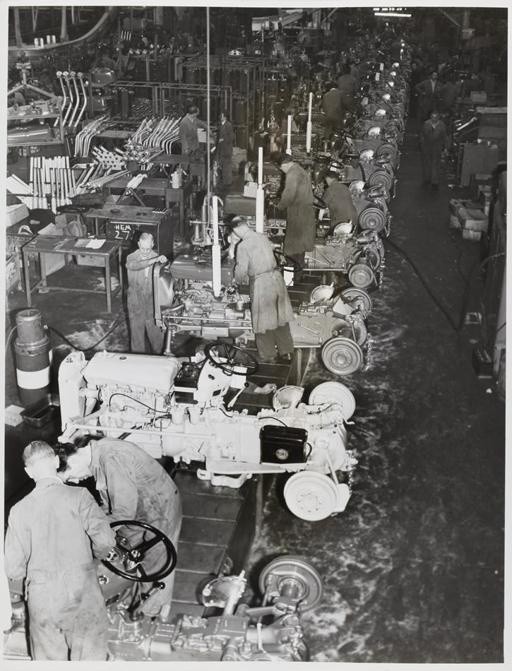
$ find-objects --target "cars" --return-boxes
[226,37,420,229]
[151,276,372,374]
[4,519,323,662]
[49,341,359,523]
[169,206,394,292]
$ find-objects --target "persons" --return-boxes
[178,105,209,156]
[125,232,168,355]
[4,440,118,662]
[319,20,449,187]
[210,109,236,186]
[230,215,296,362]
[51,436,185,623]
[320,171,360,236]
[276,152,317,282]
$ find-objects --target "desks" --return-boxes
[90,203,174,264]
[23,234,118,316]
[149,152,210,190]
[106,172,197,235]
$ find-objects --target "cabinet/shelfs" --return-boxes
[7,103,60,153]
